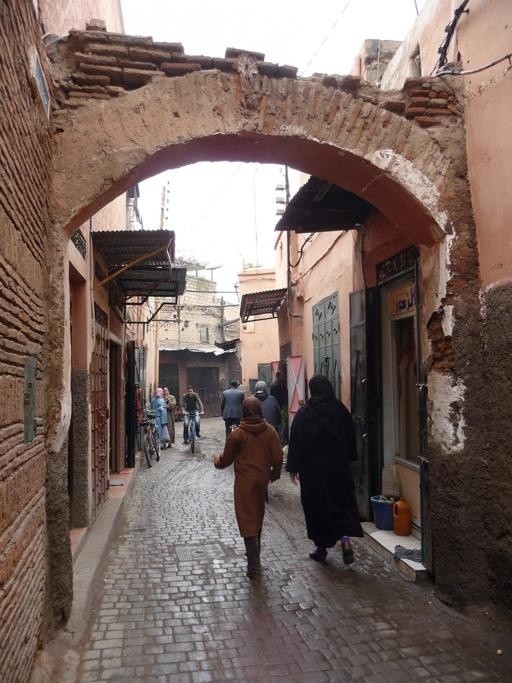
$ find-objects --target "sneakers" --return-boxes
[184,434,201,444]
[246,569,261,581]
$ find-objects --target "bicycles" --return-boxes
[182,410,207,454]
[140,408,161,467]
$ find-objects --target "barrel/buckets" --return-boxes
[393,496,411,536]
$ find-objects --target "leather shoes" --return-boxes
[342,540,354,565]
[309,548,328,562]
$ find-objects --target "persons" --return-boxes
[179,385,205,445]
[285,375,365,565]
[150,386,177,450]
[213,396,284,577]
[220,370,287,440]
